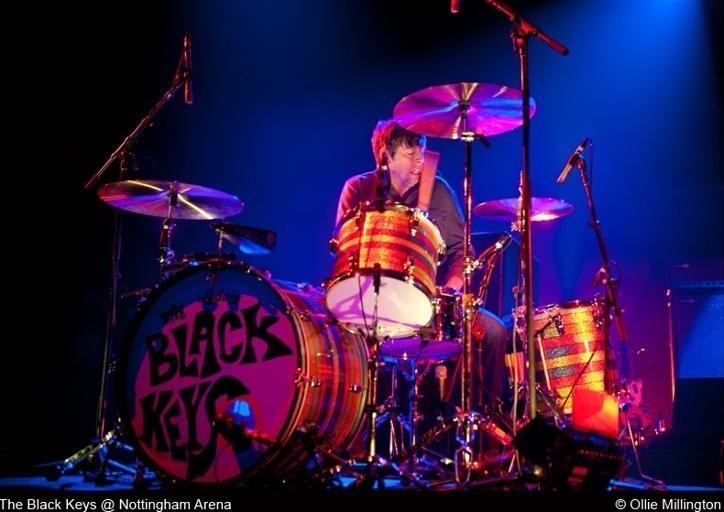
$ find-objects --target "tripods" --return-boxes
[312,356,430,489]
[463,2,661,491]
[386,135,509,489]
[31,146,152,483]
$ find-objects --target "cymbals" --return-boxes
[474,197,573,223]
[96,180,244,221]
[392,82,537,141]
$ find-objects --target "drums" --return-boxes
[377,285,464,360]
[511,298,630,422]
[163,250,240,277]
[111,258,379,487]
[324,197,447,342]
[504,326,525,401]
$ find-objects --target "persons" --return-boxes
[336,116,509,415]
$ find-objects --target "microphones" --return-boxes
[557,140,586,183]
[473,235,512,270]
[182,34,197,103]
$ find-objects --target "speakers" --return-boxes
[668,292,724,479]
